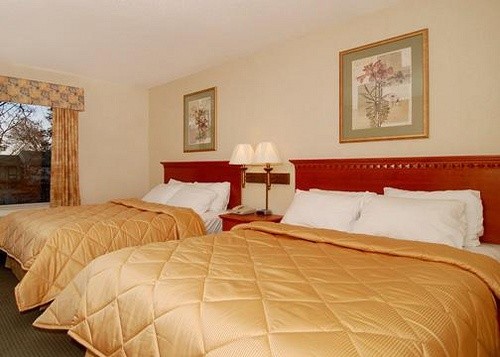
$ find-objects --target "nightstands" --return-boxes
[218,214,283,231]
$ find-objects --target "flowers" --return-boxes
[357,60,403,127]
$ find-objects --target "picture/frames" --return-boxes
[183,86,217,153]
[338,28,428,143]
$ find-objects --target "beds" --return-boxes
[1,161,242,311]
[32,155,500,357]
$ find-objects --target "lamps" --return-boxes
[229,144,255,188]
[251,142,280,191]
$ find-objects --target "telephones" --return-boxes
[224,204,256,215]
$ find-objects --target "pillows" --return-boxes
[167,178,197,186]
[194,182,231,213]
[308,189,376,195]
[168,186,217,214]
[383,187,484,248]
[353,195,469,251]
[280,188,364,231]
[142,184,182,205]
